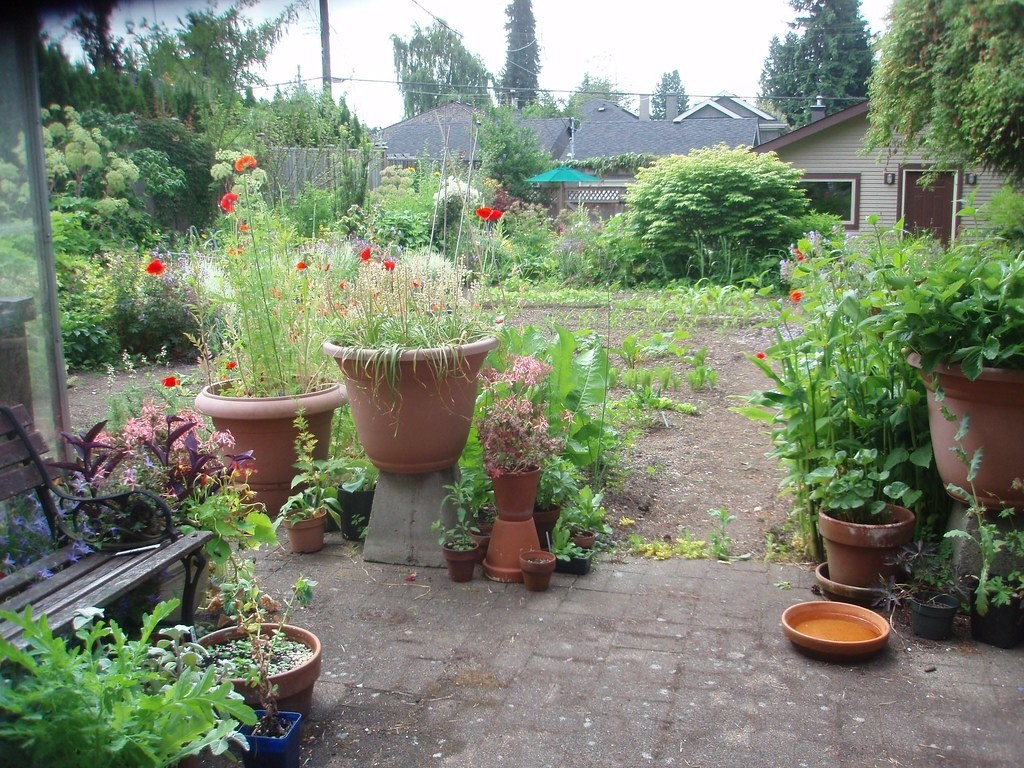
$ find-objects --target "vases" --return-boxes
[191,373,347,525]
[520,549,557,590]
[489,464,541,519]
[321,330,500,479]
[911,589,961,641]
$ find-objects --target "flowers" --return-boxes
[145,151,345,397]
[471,351,566,477]
[291,101,510,433]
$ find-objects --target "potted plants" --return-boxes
[426,473,480,584]
[942,476,1024,650]
[197,571,321,717]
[273,384,365,557]
[535,454,578,550]
[862,181,1024,513]
[570,485,614,547]
[549,516,595,576]
[338,441,378,544]
[808,447,920,584]
[322,476,341,532]
[195,568,319,768]
[464,473,496,566]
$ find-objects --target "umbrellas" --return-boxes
[522,164,603,210]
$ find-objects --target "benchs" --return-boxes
[0,402,216,668]
